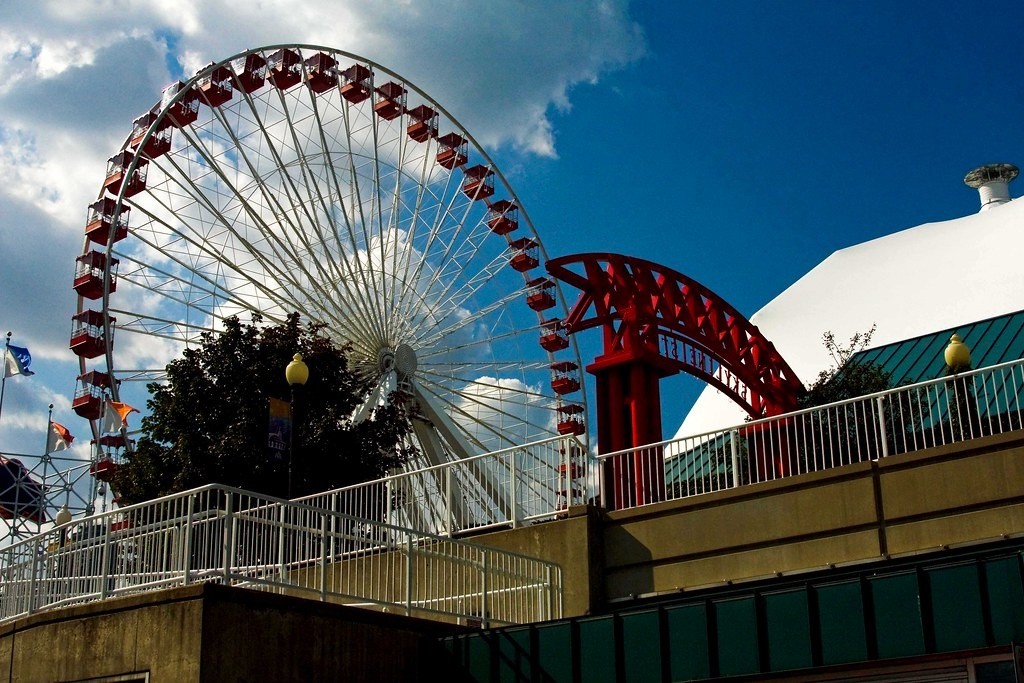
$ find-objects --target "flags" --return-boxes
[2,345,35,379]
[48,421,75,454]
[103,397,141,434]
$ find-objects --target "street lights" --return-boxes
[285,353,310,498]
[55,505,73,584]
